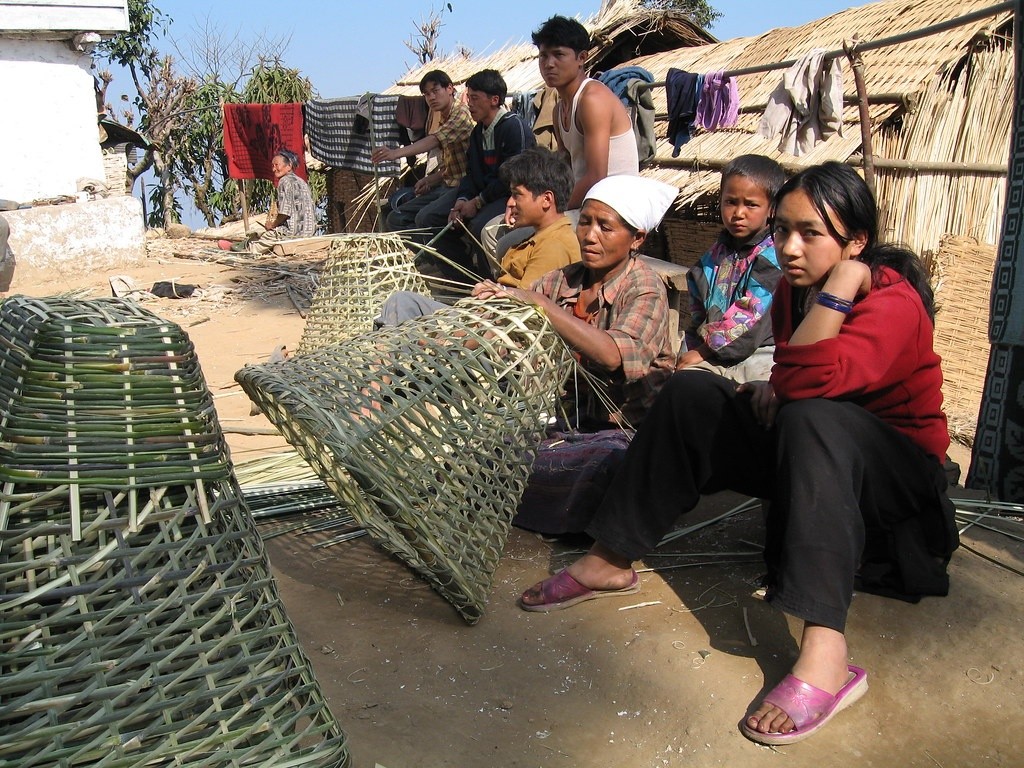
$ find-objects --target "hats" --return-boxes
[581,175,679,235]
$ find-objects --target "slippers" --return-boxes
[741,664,870,745]
[520,559,641,613]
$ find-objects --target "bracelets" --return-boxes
[814,292,853,313]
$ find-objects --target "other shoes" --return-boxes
[427,268,447,279]
[218,240,234,251]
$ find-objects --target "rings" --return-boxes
[380,151,382,154]
[771,391,777,397]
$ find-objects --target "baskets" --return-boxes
[296,230,437,390]
[234,279,577,628]
[0,289,348,768]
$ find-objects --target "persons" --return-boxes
[347,143,582,426]
[219,149,316,257]
[656,154,784,383]
[472,167,693,431]
[517,160,960,744]
[482,13,640,280]
[370,69,540,281]
